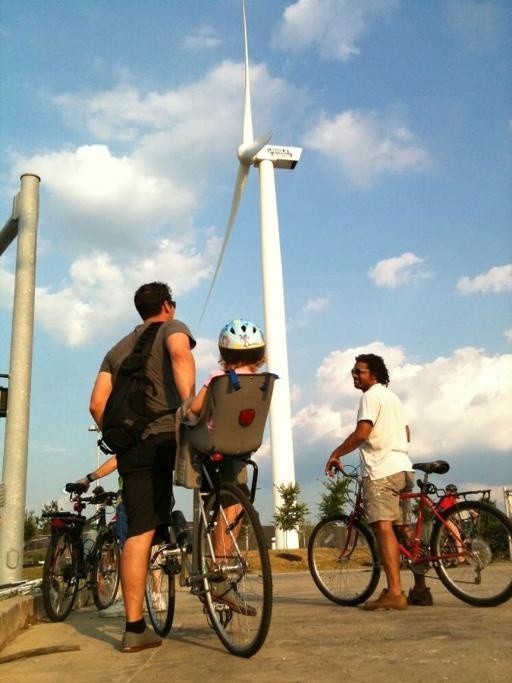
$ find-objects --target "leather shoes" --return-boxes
[122,625,162,653]
[200,576,258,617]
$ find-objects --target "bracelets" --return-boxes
[87,473,93,482]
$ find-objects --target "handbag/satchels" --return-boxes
[95,321,175,456]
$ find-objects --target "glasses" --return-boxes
[170,301,176,308]
[351,368,374,375]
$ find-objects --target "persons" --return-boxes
[75,454,167,613]
[437,483,479,565]
[324,351,434,612]
[173,317,268,486]
[86,280,259,654]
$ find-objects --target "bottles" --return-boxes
[83,524,99,556]
[170,510,193,554]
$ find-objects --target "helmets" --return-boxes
[446,484,457,491]
[217,319,267,365]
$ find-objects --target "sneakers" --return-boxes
[365,587,408,611]
[99,596,126,618]
[407,586,433,607]
[143,595,166,613]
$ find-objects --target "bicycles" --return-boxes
[42,486,120,622]
[307,459,512,607]
[87,423,281,658]
